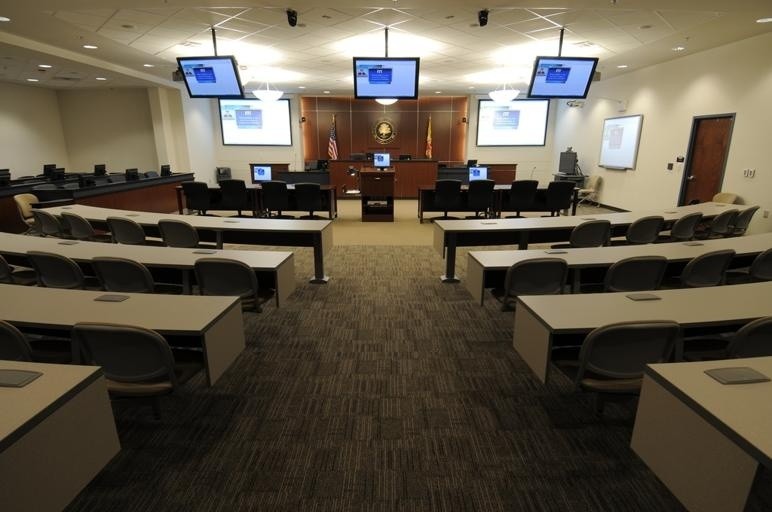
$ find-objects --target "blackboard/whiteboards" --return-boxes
[598,114,643,171]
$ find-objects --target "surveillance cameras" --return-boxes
[287,13,297,26]
[478,11,489,27]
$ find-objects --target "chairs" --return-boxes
[713,193,739,204]
[578,177,604,207]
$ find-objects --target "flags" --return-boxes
[328,116,338,160]
[425,116,432,159]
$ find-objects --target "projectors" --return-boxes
[171,71,182,82]
[591,72,602,82]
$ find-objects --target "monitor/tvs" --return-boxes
[558,151,577,174]
[468,166,488,182]
[252,164,272,184]
[527,56,599,99]
[352,56,420,99]
[0,164,171,187]
[373,152,391,170]
[318,160,328,169]
[351,153,367,160]
[467,160,477,167]
[176,56,243,100]
[398,155,411,161]
